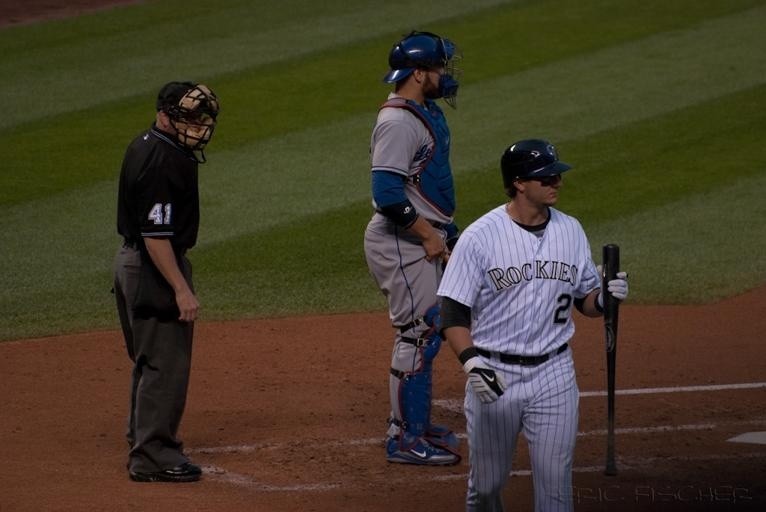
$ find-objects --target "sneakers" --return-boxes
[383,436,462,467]
[128,460,202,481]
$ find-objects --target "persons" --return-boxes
[109,75,219,483]
[430,134,627,512]
[360,29,463,469]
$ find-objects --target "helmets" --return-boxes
[381,33,455,86]
[500,138,574,187]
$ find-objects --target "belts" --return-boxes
[375,200,449,233]
[479,341,569,369]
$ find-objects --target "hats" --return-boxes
[156,82,218,118]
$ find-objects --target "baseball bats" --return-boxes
[602,244,620,470]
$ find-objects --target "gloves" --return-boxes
[594,263,632,316]
[457,346,509,406]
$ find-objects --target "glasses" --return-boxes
[524,172,561,185]
